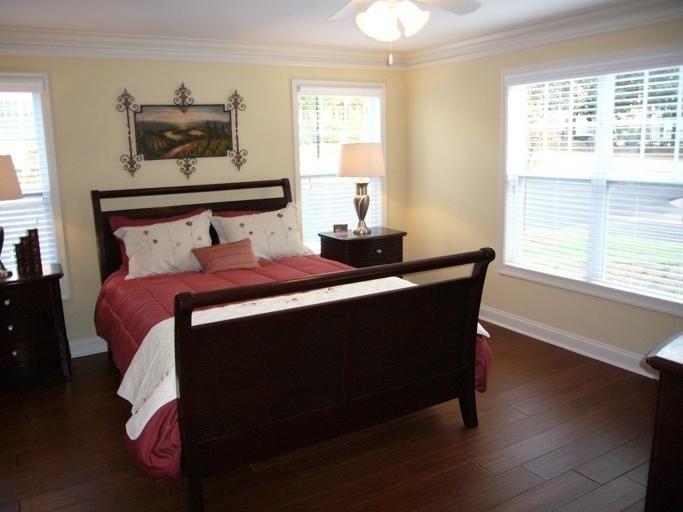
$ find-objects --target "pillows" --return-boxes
[109,200,315,281]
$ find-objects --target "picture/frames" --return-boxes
[115,82,248,178]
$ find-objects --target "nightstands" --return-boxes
[0,263,73,390]
[319,226,407,279]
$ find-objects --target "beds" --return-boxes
[91,179,495,512]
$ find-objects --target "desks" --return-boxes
[645,332,683,512]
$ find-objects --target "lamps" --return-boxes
[341,144,385,234]
[356,0,431,66]
[0,155,24,278]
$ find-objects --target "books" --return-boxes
[18,235,33,276]
[15,243,26,276]
[27,228,42,276]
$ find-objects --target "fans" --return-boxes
[330,0,482,22]
